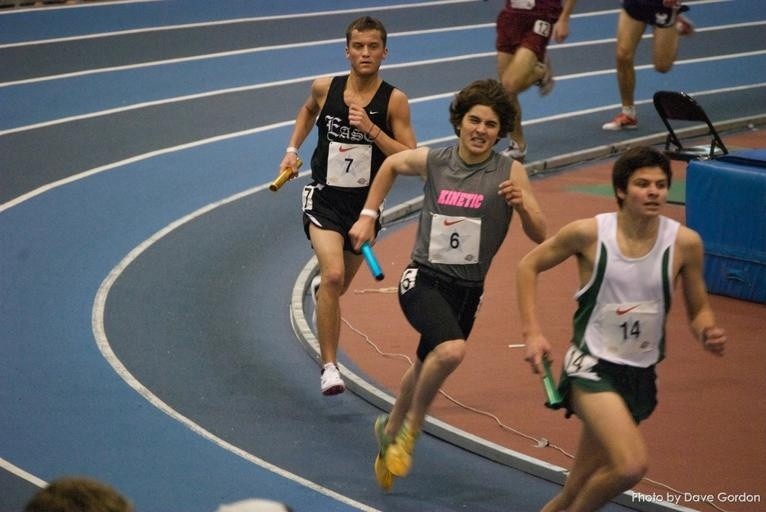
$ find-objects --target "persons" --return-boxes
[279,14,420,396]
[349,78,549,493]
[25,475,135,512]
[514,142,729,512]
[216,497,295,512]
[602,0,696,131]
[488,0,577,158]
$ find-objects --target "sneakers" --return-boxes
[319,362,345,395]
[499,140,528,163]
[675,15,695,36]
[601,112,638,130]
[311,275,321,336]
[373,414,421,493]
[537,56,554,96]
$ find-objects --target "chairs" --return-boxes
[652,89,730,165]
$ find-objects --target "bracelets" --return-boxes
[366,122,375,134]
[372,128,382,141]
[286,147,299,154]
[360,207,380,219]
